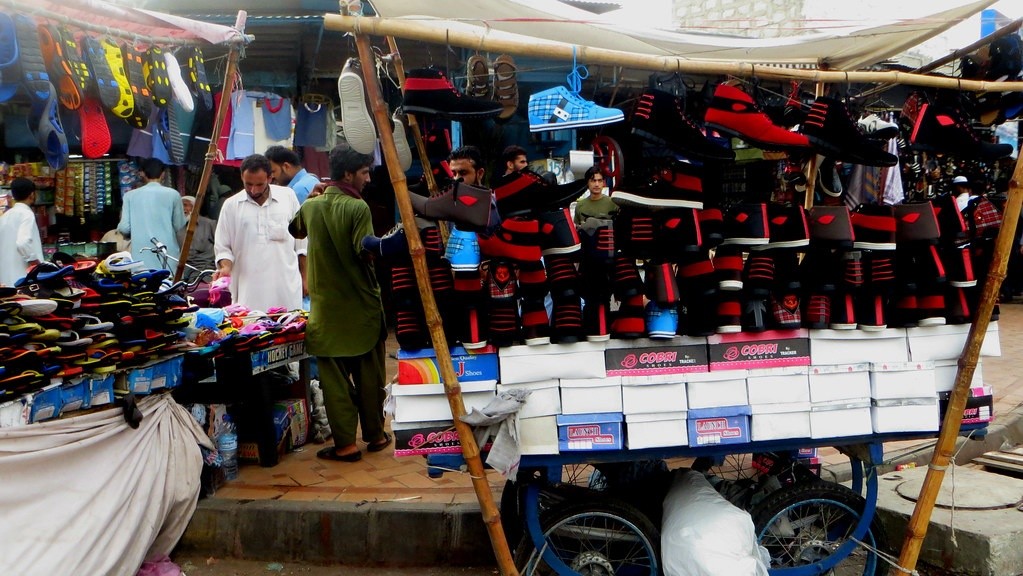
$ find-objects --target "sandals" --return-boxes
[961,54,1004,126]
[491,54,521,122]
[989,34,1023,120]
[467,55,489,100]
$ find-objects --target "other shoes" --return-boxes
[392,112,413,172]
[338,57,377,155]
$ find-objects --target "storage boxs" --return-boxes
[43,243,117,260]
[0,340,310,465]
[390,322,1001,456]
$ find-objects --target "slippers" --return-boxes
[31,80,69,170]
[0,250,199,403]
[366,431,392,451]
[0,10,223,159]
[316,446,361,461]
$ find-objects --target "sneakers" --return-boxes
[631,88,736,163]
[528,86,625,132]
[401,68,504,121]
[778,88,1014,166]
[363,160,1001,352]
[704,82,810,152]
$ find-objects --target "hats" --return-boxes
[953,176,968,183]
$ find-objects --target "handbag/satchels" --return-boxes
[293,101,328,146]
[213,92,243,169]
[314,98,337,153]
[226,89,295,162]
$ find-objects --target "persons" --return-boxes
[217,145,617,462]
[116,159,187,279]
[179,195,218,285]
[0,176,44,287]
[952,174,1012,211]
